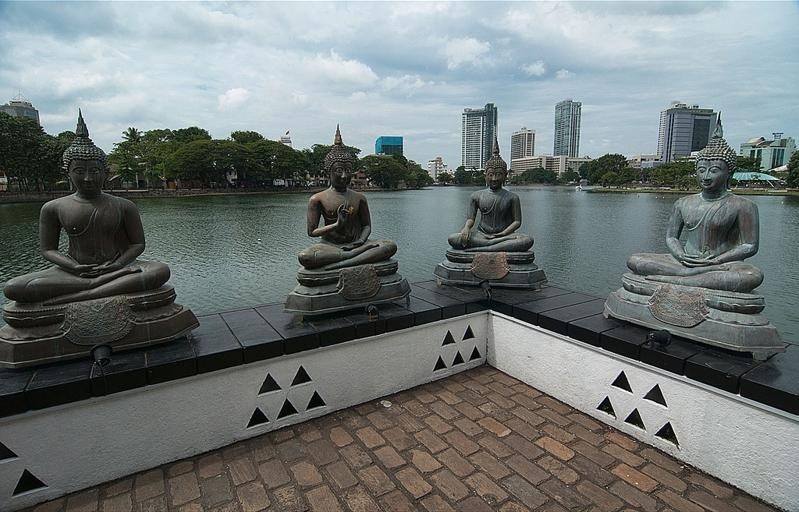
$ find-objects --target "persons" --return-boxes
[626,139,764,293]
[448,156,533,252]
[3,138,170,306]
[298,148,397,270]
